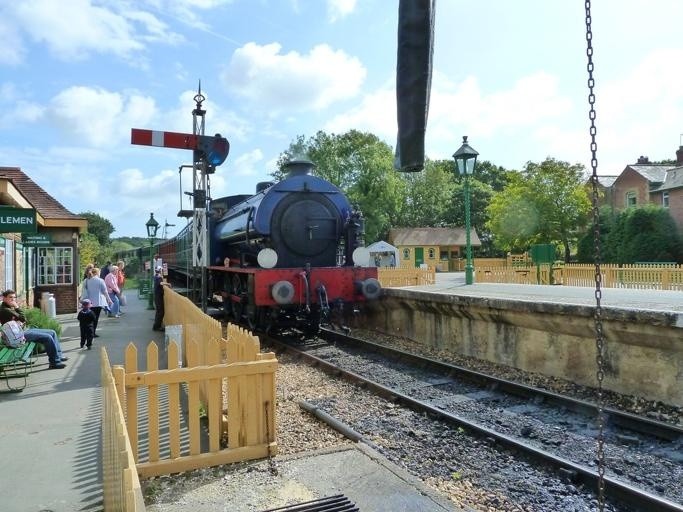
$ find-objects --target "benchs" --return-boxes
[0,323,36,392]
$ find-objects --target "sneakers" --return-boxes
[49,356,68,369]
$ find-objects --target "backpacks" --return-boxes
[2,321,26,348]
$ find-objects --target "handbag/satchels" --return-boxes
[78,284,88,302]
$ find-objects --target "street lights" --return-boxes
[452,135,479,286]
[145,212,158,309]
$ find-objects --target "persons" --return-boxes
[374,252,381,266]
[0,290,70,369]
[152,266,171,331]
[77,260,125,350]
[388,253,394,267]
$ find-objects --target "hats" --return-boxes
[80,299,93,308]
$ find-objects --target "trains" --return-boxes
[110,160,382,347]
